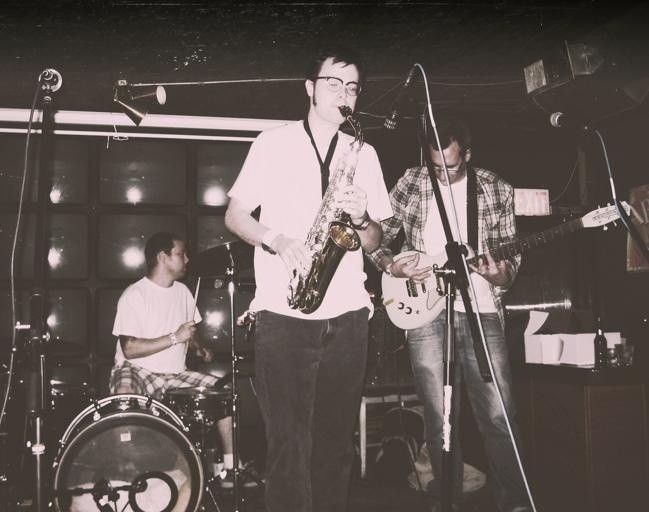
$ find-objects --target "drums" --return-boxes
[51,393,206,512]
[164,385,238,425]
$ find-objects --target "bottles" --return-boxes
[594,315,608,368]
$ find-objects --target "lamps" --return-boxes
[113,85,168,128]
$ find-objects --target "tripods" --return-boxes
[204,293,264,511]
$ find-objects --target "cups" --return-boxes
[608,344,633,369]
[540,338,563,362]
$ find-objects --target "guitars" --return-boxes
[381,201,631,330]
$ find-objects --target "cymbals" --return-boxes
[195,241,254,273]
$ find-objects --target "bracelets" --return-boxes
[169,330,180,350]
[258,229,284,254]
[385,263,393,277]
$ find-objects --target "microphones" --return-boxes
[550,112,593,136]
[39,69,63,92]
[383,65,414,130]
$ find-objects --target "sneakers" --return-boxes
[220,465,266,488]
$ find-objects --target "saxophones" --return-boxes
[287,105,364,314]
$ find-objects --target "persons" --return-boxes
[364,100,539,512]
[223,52,384,511]
[105,231,247,490]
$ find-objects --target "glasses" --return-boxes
[314,77,363,97]
[433,157,463,175]
